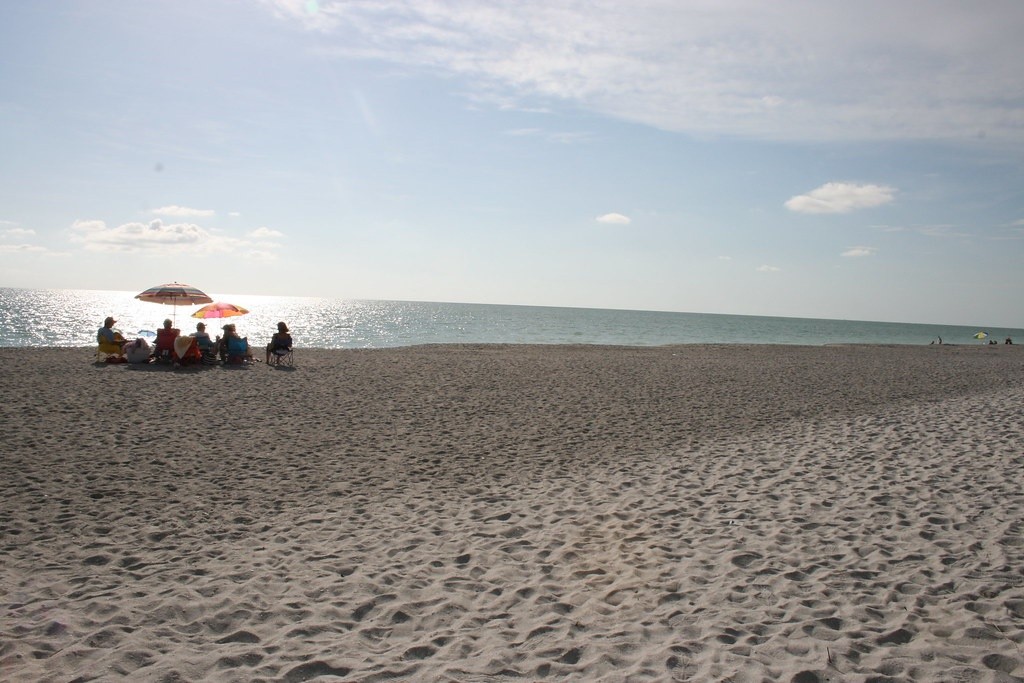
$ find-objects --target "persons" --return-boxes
[153,319,172,356]
[266,321,292,364]
[97,317,131,347]
[189,322,216,357]
[219,324,251,363]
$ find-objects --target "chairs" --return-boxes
[268,338,293,366]
[154,328,248,366]
[97,335,130,361]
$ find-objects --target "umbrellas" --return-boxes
[191,303,249,339]
[134,282,214,329]
[137,329,157,337]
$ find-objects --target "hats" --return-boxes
[163,319,172,325]
[196,322,206,328]
[104,317,117,323]
